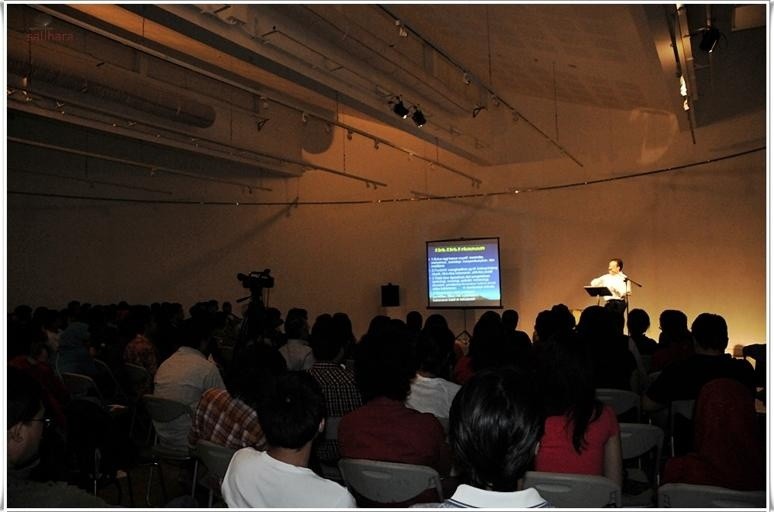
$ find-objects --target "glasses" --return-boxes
[24,417,51,431]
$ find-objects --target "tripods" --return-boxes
[454,310,471,341]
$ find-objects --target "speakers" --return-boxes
[381,283,400,308]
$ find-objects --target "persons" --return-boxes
[589,257,632,313]
[5,298,767,510]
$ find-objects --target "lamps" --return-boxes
[387,95,427,129]
